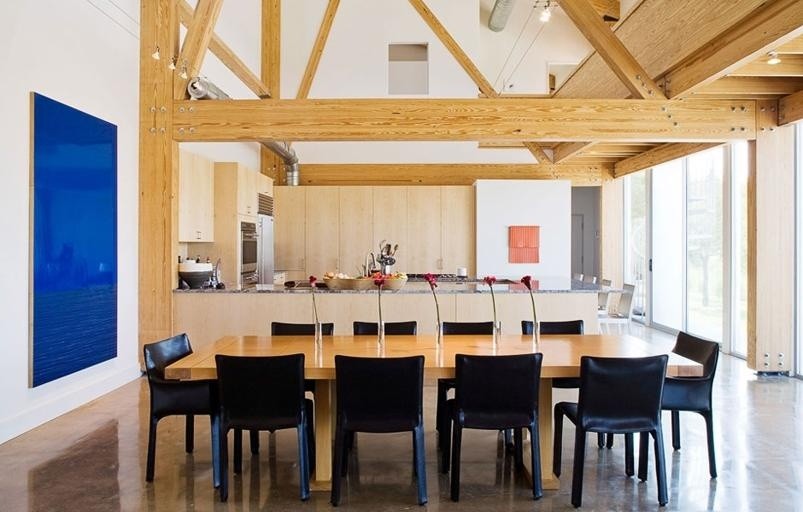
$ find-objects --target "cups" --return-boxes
[455,266,467,278]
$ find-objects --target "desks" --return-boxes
[163,334,706,491]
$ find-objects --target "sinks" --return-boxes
[293,281,328,288]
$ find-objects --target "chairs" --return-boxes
[606,330,719,481]
[215,352,315,502]
[329,354,427,507]
[573,271,636,335]
[436,321,503,432]
[353,320,417,335]
[440,352,543,502]
[553,354,669,507]
[143,332,259,488]
[271,322,335,335]
[521,319,606,450]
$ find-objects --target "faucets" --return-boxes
[364,251,376,277]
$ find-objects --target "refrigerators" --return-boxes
[257,216,274,286]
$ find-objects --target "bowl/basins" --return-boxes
[321,277,408,293]
[178,262,212,289]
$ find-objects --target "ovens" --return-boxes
[240,221,257,275]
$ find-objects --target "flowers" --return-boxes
[309,275,321,347]
[422,273,441,345]
[520,275,538,342]
[482,276,498,343]
[372,272,392,343]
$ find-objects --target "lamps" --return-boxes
[766,51,781,66]
[540,1,551,21]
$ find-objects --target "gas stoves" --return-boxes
[406,273,469,282]
